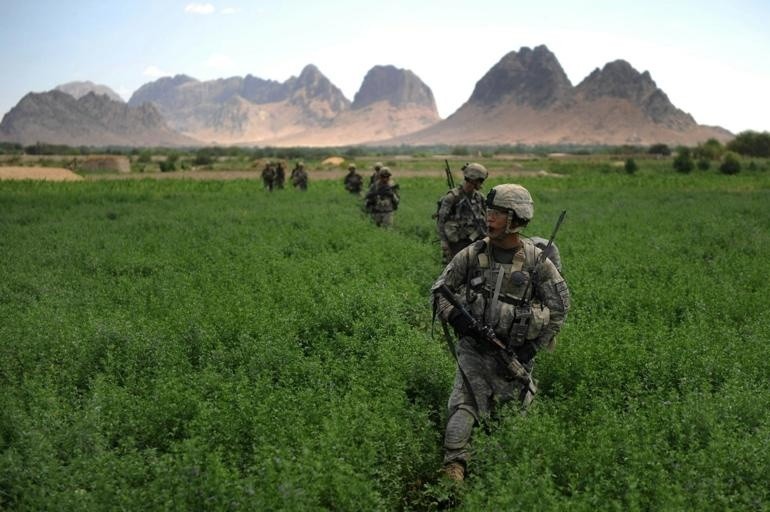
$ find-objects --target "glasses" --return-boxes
[486,208,503,216]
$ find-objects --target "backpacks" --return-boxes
[468,236,562,272]
[431,188,458,224]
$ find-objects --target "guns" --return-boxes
[439,285,536,396]
[360,184,400,200]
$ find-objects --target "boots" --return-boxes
[443,461,466,482]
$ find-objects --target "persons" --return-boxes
[437,162,489,266]
[343,161,400,227]
[432,184,569,481]
[260,160,309,193]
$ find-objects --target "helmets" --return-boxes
[348,162,392,177]
[487,184,534,221]
[462,163,487,179]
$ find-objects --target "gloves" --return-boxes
[503,346,536,382]
[453,315,491,349]
